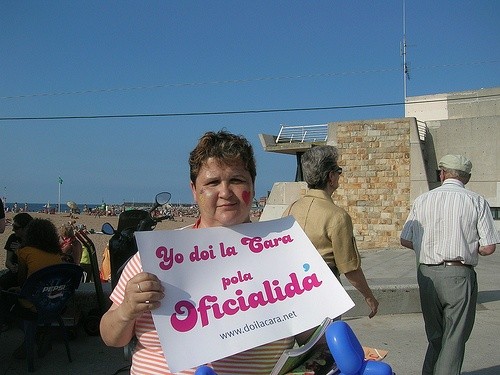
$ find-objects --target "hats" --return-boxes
[439,154,472,173]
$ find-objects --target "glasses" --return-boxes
[12,224,22,230]
[336,168,342,175]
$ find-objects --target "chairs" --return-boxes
[0,263,84,373]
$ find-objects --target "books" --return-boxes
[270,316,332,375]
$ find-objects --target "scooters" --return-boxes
[102,191,172,297]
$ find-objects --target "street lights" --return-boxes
[56,175,64,213]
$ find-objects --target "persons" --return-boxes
[14,202,126,218]
[400,155,500,375]
[0,219,67,332]
[0,213,35,312]
[61,226,82,263]
[280,146,379,328]
[100,128,331,375]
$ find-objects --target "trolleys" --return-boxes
[75,229,109,337]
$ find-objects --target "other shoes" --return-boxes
[12,344,27,359]
[38,335,52,357]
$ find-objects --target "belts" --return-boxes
[442,261,470,266]
